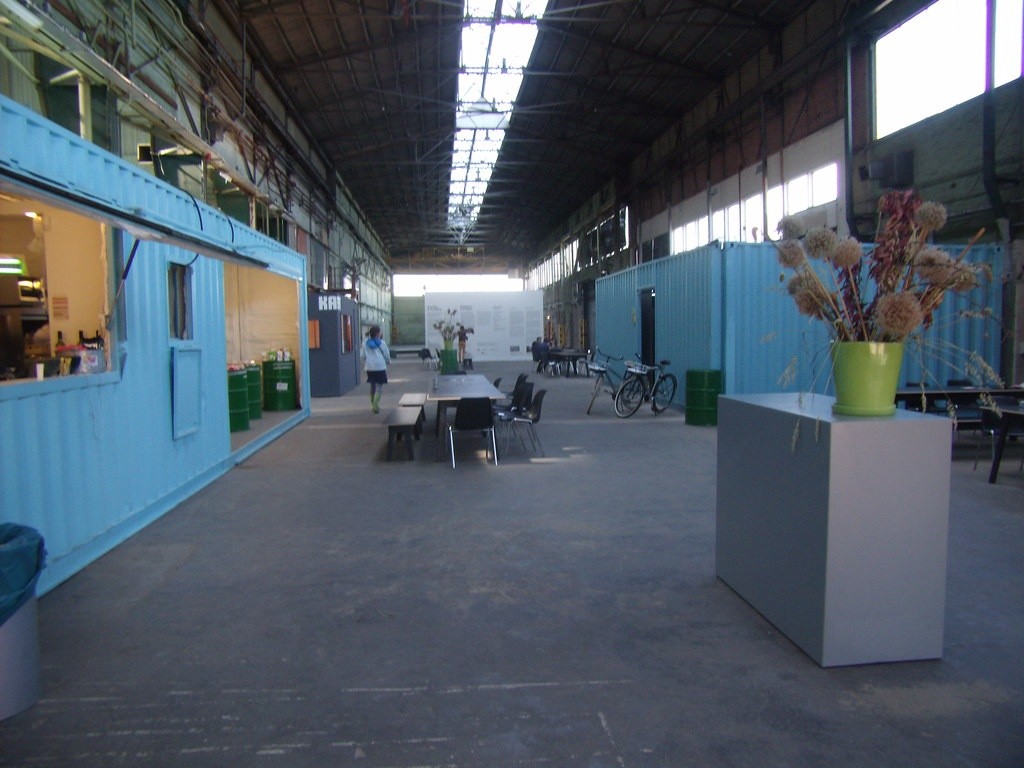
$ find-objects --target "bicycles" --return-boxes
[614,352,678,418]
[585,346,645,415]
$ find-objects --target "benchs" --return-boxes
[382,392,426,461]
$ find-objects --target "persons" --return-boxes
[532,337,558,372]
[360,325,392,414]
[458,326,468,363]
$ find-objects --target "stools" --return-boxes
[463,358,473,370]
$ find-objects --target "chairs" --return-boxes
[446,373,546,468]
[417,348,440,369]
[894,379,1024,471]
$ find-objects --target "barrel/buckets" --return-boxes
[684,369,721,427]
[245,366,261,420]
[262,360,298,412]
[228,369,250,433]
[441,349,457,373]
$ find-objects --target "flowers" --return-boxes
[760,188,1006,452]
[434,309,474,341]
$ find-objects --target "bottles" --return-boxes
[278,349,283,361]
[56,330,65,357]
[433,370,439,390]
[228,361,239,371]
[77,330,85,346]
[284,349,291,361]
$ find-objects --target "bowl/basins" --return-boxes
[25,359,59,378]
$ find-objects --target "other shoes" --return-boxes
[372,405,379,413]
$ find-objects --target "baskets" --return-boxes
[626,359,648,375]
[585,357,608,371]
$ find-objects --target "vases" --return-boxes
[444,340,454,350]
[830,341,905,418]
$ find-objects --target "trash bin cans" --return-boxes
[0,521,46,725]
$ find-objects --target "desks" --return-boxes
[716,392,953,668]
[896,386,1024,440]
[548,352,589,378]
[427,375,506,462]
[980,405,1024,484]
